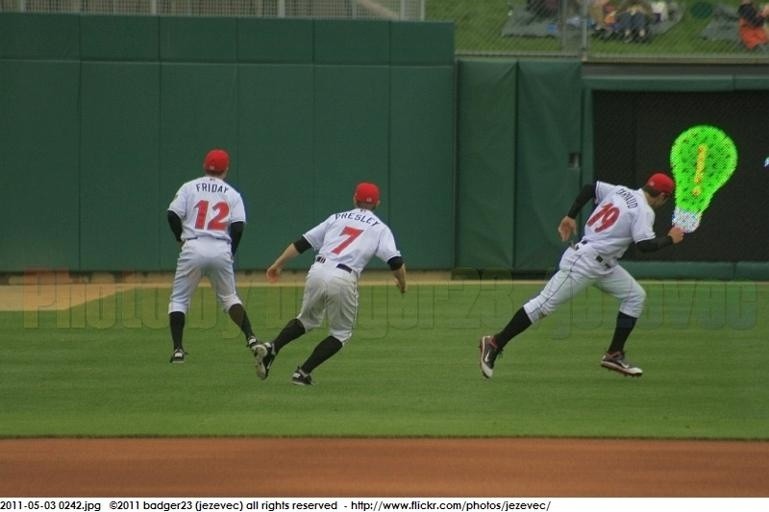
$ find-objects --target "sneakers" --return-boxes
[248,337,275,380]
[291,368,313,384]
[480,335,498,379]
[170,347,185,363]
[601,351,642,376]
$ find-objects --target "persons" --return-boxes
[167,148,262,365]
[480,172,684,378]
[255,183,406,386]
[526,0,769,53]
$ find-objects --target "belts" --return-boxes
[581,239,609,267]
[316,256,351,271]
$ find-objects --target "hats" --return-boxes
[202,149,229,173]
[645,174,673,194]
[355,182,380,204]
